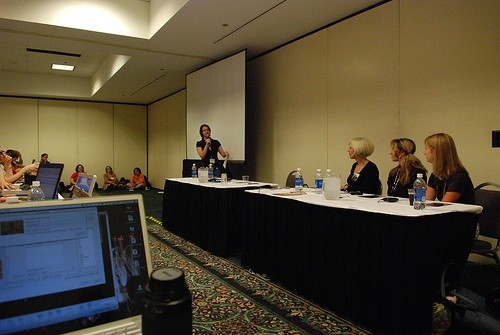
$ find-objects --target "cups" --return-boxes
[242,175,249,185]
[221,173,227,186]
[14,184,19,190]
[408,189,413,206]
[6,197,18,202]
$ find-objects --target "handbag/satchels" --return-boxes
[214,164,232,177]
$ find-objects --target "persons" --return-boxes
[40,154,50,163]
[0,150,39,203]
[69,164,85,197]
[59,181,101,192]
[387,137,426,201]
[128,167,148,191]
[339,137,381,201]
[119,176,130,188]
[196,124,229,178]
[102,166,121,192]
[425,132,474,205]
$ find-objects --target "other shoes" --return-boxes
[446,296,457,304]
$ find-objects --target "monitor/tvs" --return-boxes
[35,163,64,199]
[72,172,97,198]
[0,193,154,335]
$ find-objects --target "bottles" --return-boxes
[208,164,214,179]
[295,168,304,191]
[314,169,323,194]
[192,164,197,178]
[323,169,332,193]
[413,173,427,210]
[27,181,45,201]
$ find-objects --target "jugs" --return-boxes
[323,174,341,199]
[198,166,208,184]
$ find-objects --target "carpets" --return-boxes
[146,192,376,335]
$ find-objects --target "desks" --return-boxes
[161,176,279,261]
[242,186,484,335]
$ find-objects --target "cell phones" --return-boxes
[380,198,399,202]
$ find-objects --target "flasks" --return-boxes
[142,267,192,335]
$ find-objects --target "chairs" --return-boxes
[285,170,303,188]
[456,181,500,304]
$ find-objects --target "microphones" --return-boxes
[207,133,210,146]
